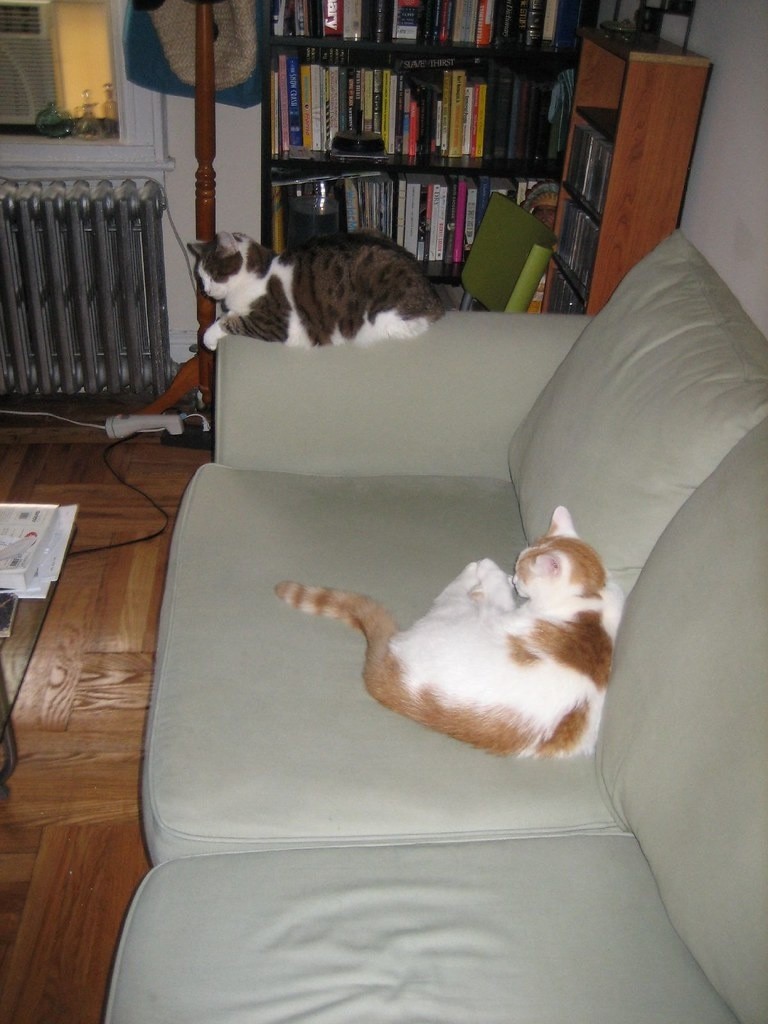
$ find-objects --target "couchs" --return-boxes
[90,228,768,1024]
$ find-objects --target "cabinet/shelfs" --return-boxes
[259,0,600,283]
[534,30,709,316]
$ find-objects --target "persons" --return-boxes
[522,184,561,316]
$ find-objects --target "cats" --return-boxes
[276,500,624,761]
[183,231,445,352]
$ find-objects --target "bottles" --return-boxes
[74,87,104,140]
[35,99,74,138]
[101,84,118,138]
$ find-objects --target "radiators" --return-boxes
[0,177,204,411]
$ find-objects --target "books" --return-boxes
[548,270,583,316]
[270,47,568,160]
[0,503,78,637]
[555,199,601,287]
[272,0,580,46]
[271,167,556,264]
[566,124,614,214]
